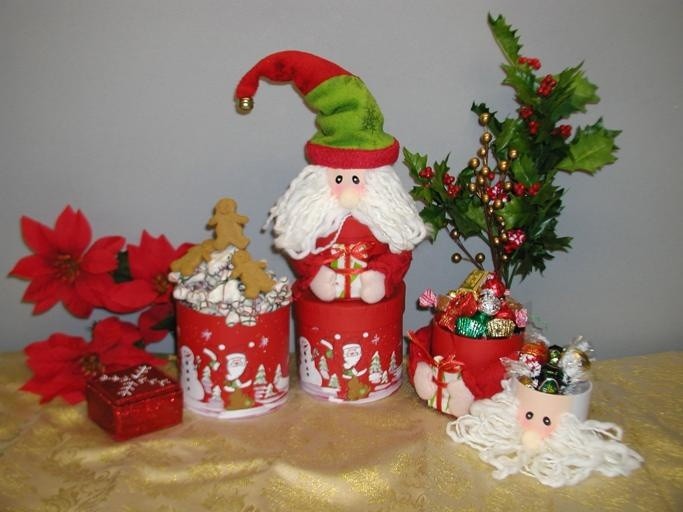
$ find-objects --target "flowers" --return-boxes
[5,208,198,409]
[398,6,623,300]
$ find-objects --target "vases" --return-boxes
[423,321,531,375]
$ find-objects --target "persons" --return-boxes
[236,50,426,306]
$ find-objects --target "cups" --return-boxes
[408,315,547,423]
[508,367,594,443]
[292,277,406,404]
[172,284,291,422]
